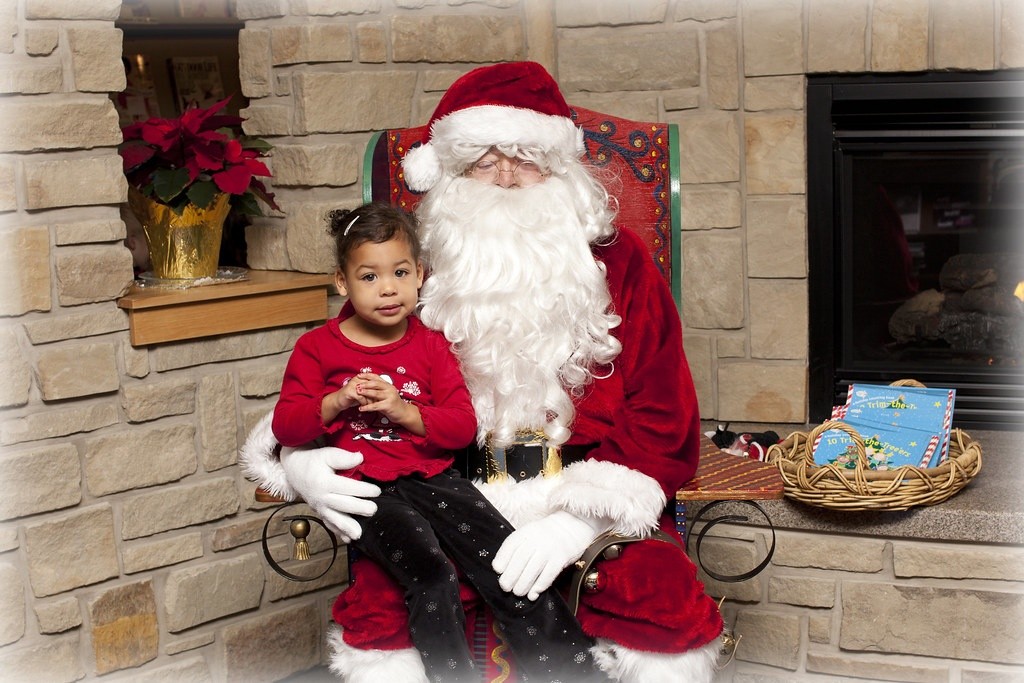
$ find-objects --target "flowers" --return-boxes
[118,88,286,213]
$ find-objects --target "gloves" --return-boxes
[280,444,382,541]
[491,515,612,603]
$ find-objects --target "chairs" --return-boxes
[254,104,784,683]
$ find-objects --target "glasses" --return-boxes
[468,160,542,190]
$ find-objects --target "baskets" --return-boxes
[767,376,983,512]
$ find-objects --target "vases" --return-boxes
[128,192,232,280]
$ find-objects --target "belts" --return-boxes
[450,443,600,482]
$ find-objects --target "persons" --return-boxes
[240,60,727,683]
[272,204,615,683]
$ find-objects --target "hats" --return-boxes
[401,59,586,191]
